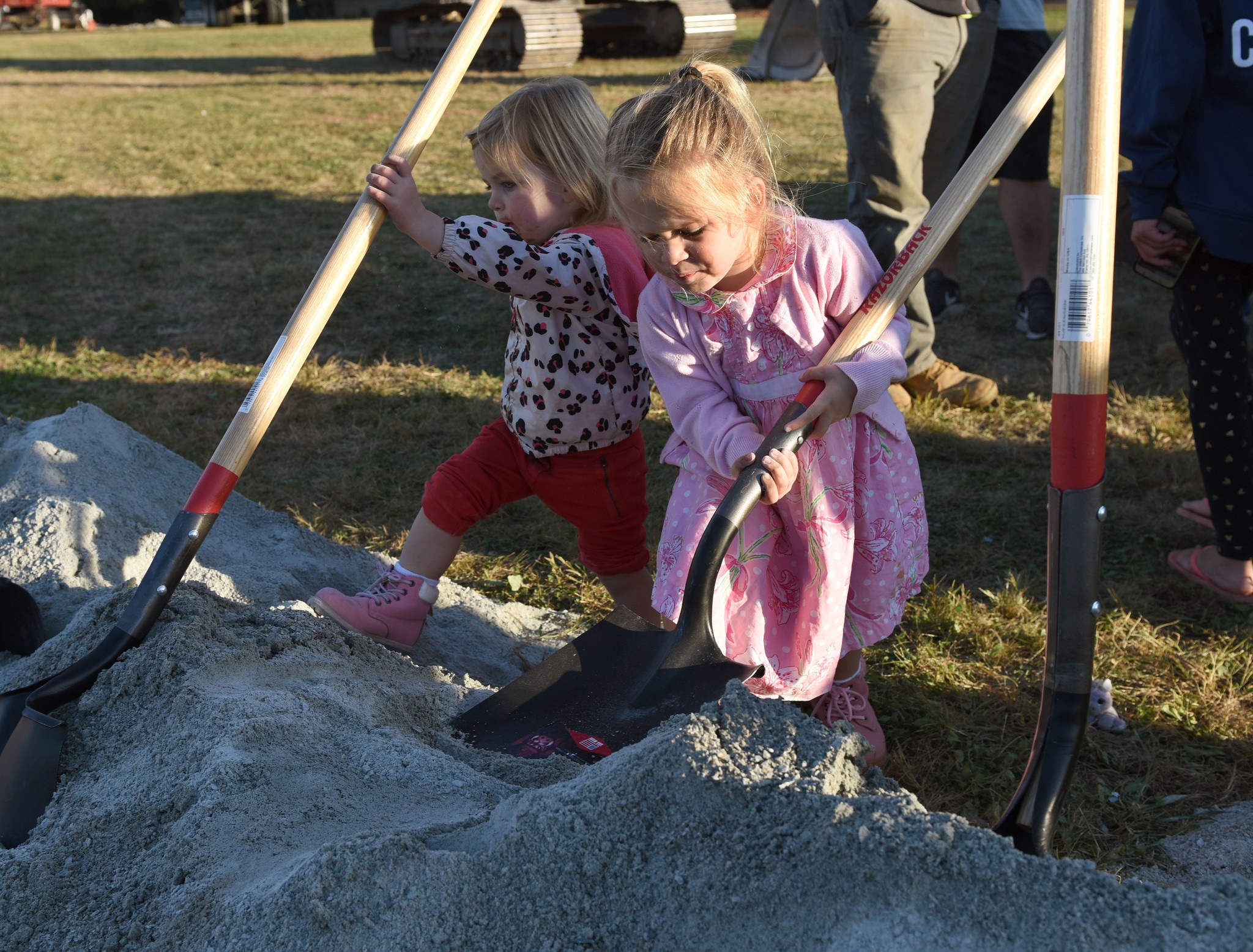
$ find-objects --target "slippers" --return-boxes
[1176,498,1215,530]
[1168,548,1253,602]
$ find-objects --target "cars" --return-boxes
[0,0,90,33]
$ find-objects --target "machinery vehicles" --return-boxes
[372,0,736,74]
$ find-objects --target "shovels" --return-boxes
[444,19,1068,766]
[0,1,493,848]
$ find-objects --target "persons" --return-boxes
[922,0,1056,341]
[604,59,931,766]
[1120,0,1253,602]
[310,75,676,654]
[814,0,1002,415]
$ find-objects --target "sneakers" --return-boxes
[910,271,968,322]
[307,562,440,651]
[1012,272,1058,340]
[810,655,885,767]
